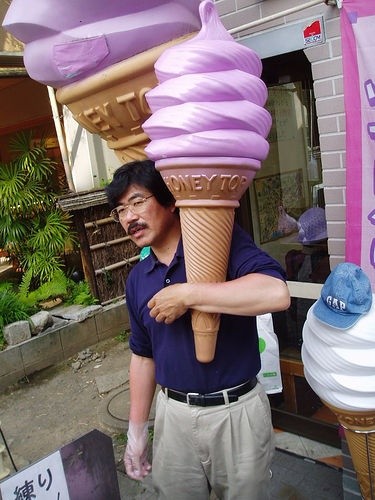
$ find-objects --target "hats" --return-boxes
[312,260,373,333]
[296,207,328,243]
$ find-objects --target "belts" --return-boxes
[157,376,261,408]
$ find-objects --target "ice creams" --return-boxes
[0,1,203,164]
[300,262,375,499]
[141,0,274,365]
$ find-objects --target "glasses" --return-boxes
[108,193,162,224]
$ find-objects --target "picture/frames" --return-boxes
[252,168,307,245]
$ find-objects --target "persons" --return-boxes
[106,158,292,500]
[282,204,330,283]
